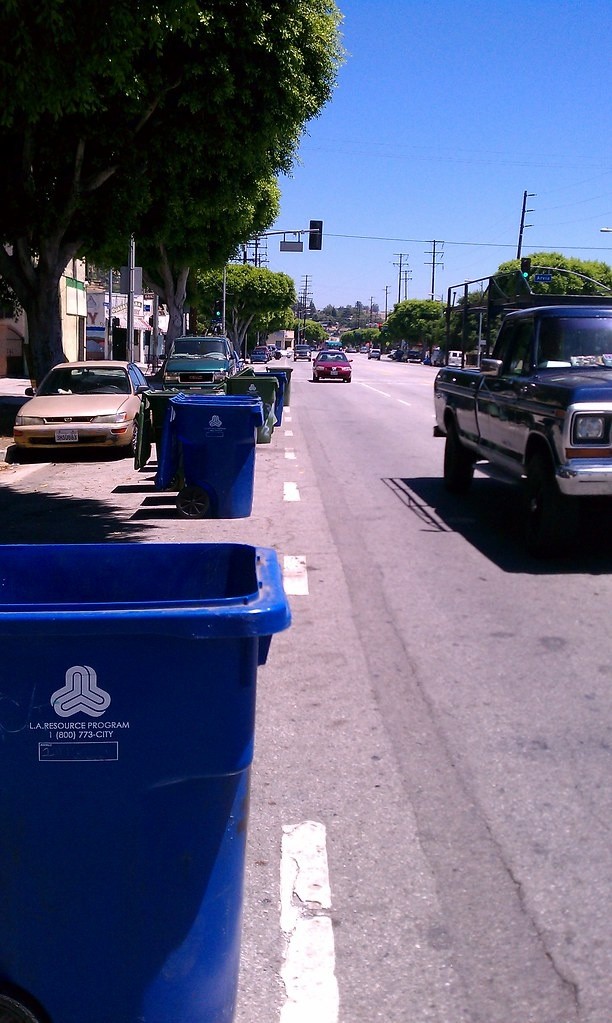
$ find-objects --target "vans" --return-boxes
[319,341,343,353]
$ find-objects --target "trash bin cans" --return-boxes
[0,543,294,1023]
[134,389,180,471]
[154,392,264,519]
[254,372,288,427]
[225,376,280,444]
[266,367,293,406]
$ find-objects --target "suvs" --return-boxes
[163,335,237,395]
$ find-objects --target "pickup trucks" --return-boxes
[431,304,612,541]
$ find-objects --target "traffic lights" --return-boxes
[214,299,222,319]
[521,258,531,282]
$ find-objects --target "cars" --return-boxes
[250,344,291,365]
[312,350,353,383]
[345,348,356,353]
[292,344,313,363]
[367,348,381,361]
[14,360,153,458]
